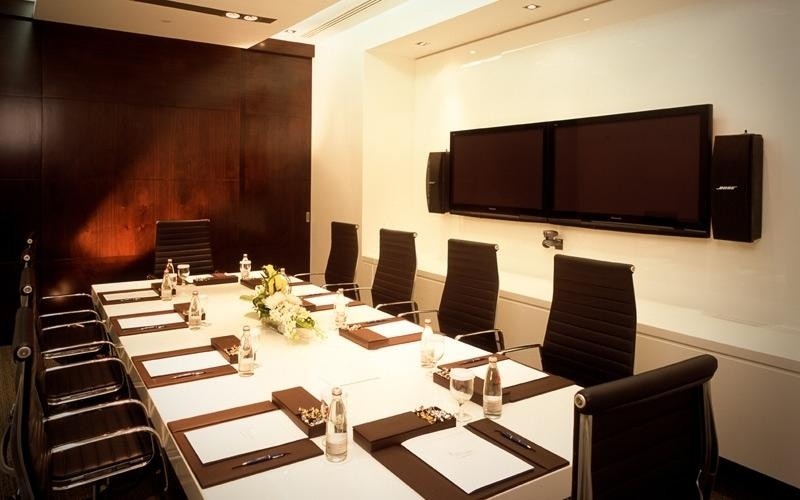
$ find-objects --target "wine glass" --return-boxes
[448,368,475,422]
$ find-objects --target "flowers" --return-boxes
[238,262,331,346]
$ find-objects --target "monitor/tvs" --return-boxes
[549,103,713,239]
[450,122,549,224]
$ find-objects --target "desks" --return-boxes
[90,266,585,500]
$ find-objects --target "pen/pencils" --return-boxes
[366,319,388,325]
[494,428,537,453]
[121,298,143,302]
[313,294,321,295]
[140,324,166,331]
[172,370,207,380]
[458,356,488,367]
[230,452,292,470]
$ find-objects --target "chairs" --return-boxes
[571,354,719,500]
[146,219,214,282]
[292,221,360,302]
[340,228,420,326]
[1,234,172,499]
[495,254,640,389]
[397,238,509,358]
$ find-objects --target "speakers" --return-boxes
[424,151,450,213]
[712,134,764,244]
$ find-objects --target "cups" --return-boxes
[178,264,190,284]
[239,261,251,279]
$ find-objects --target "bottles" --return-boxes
[236,325,255,377]
[483,355,502,421]
[280,267,289,278]
[240,253,250,261]
[188,291,203,331]
[160,258,178,299]
[334,288,348,328]
[418,318,438,369]
[325,387,349,464]
